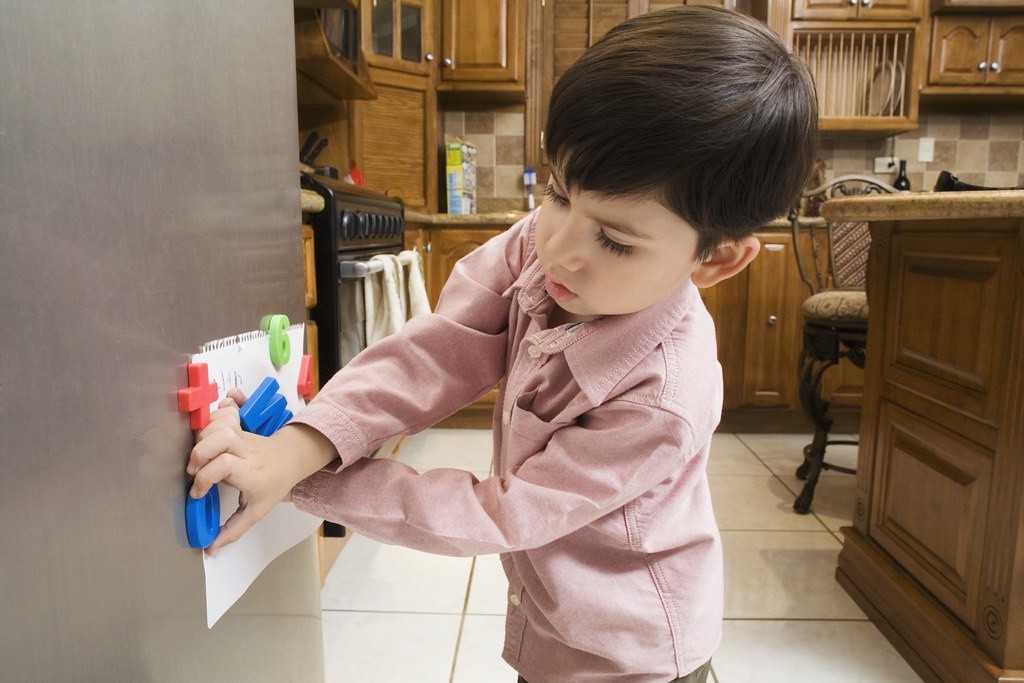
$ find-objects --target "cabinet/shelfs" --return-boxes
[406,224,870,433]
[834,218,1024,683]
[293,0,1024,210]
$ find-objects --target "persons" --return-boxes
[188,4,820,683]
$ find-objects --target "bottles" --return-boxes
[893,159,910,193]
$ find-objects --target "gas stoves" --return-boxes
[299,162,405,252]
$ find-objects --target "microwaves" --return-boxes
[317,1,358,75]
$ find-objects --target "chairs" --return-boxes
[788,173,898,514]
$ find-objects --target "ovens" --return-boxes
[314,246,422,537]
